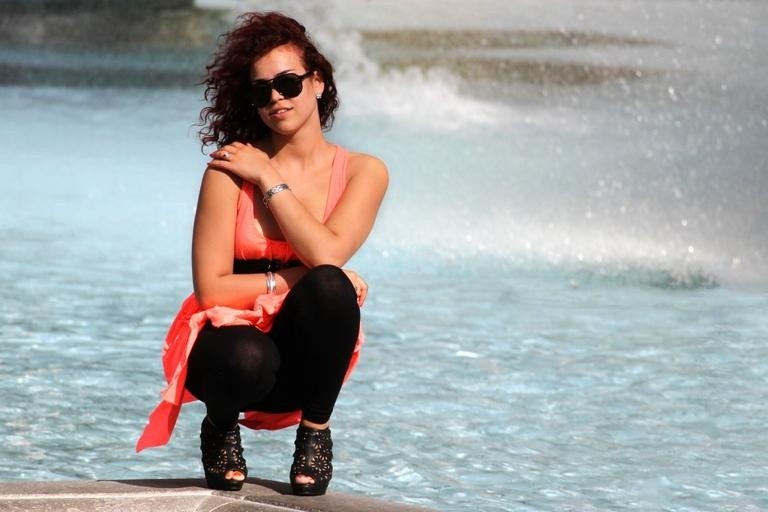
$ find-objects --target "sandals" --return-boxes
[200,417,246,491]
[290,424,331,495]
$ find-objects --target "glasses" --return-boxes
[245,72,312,107]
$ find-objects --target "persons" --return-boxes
[133,7,390,497]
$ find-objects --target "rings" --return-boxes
[222,153,230,159]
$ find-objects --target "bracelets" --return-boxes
[263,182,293,210]
[265,270,277,295]
